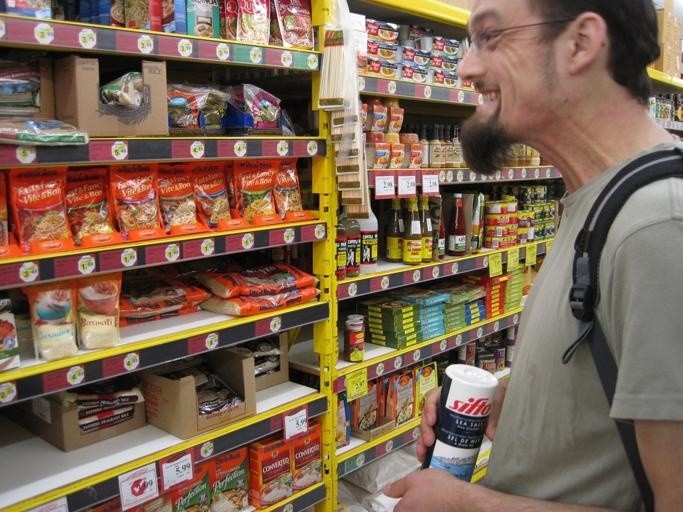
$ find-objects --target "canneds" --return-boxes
[490,184,557,245]
[484,199,520,248]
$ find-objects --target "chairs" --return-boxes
[0,0,332,512]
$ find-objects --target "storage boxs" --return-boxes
[654,45,681,79]
[144,347,256,440]
[57,55,170,136]
[1,54,57,129]
[1,393,146,452]
[656,9,681,47]
[256,331,290,392]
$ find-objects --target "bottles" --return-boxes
[446,192,466,256]
[402,196,423,266]
[336,197,347,281]
[418,195,433,263]
[338,205,362,278]
[399,122,468,169]
[438,198,446,260]
[385,198,405,263]
[338,190,378,275]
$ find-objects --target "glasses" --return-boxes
[455,17,606,60]
